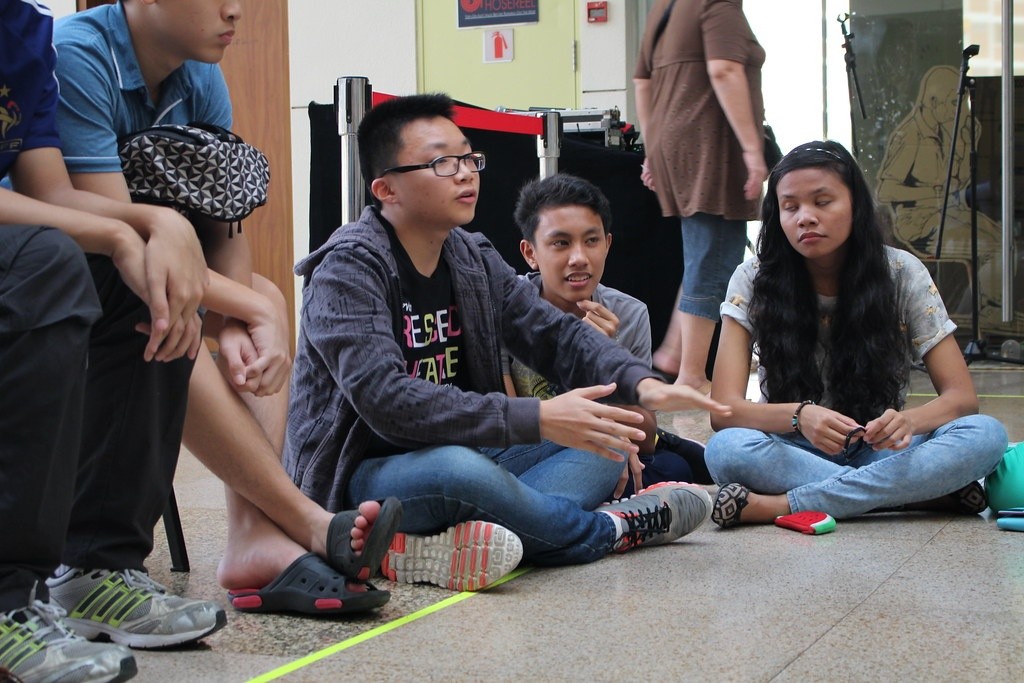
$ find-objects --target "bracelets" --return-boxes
[843,427,867,459]
[792,400,816,431]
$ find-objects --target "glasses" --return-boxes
[377,151,486,177]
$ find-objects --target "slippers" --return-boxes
[328,497,402,582]
[228,551,389,612]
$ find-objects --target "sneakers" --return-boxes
[382,520,523,592]
[0,601,137,683]
[38,566,226,649]
[593,480,713,555]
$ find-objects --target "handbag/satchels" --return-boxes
[764,124,783,171]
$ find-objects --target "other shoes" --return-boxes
[711,482,751,529]
[934,480,988,514]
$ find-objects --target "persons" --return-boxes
[632,0,768,398]
[280,93,735,591]
[704,140,1008,528]
[501,173,716,504]
[0,0,228,683]
[50,0,403,619]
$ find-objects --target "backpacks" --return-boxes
[116,118,272,238]
